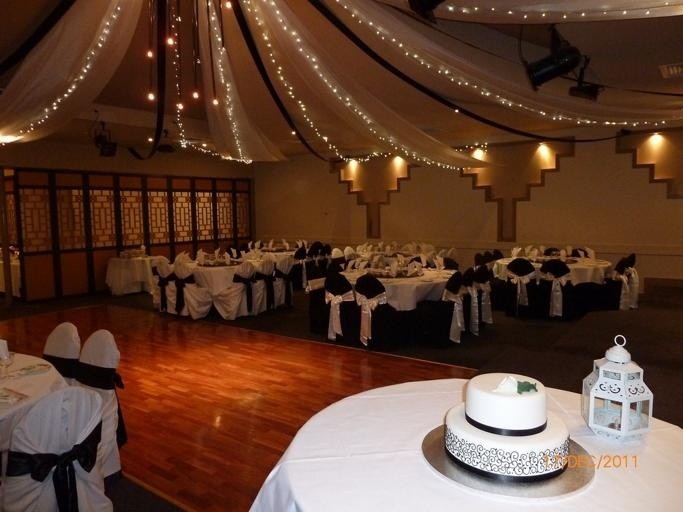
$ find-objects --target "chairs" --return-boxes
[473,244,638,319]
[307,244,489,351]
[153,241,307,322]
[0,322,128,511]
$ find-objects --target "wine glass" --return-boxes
[0,352,15,380]
